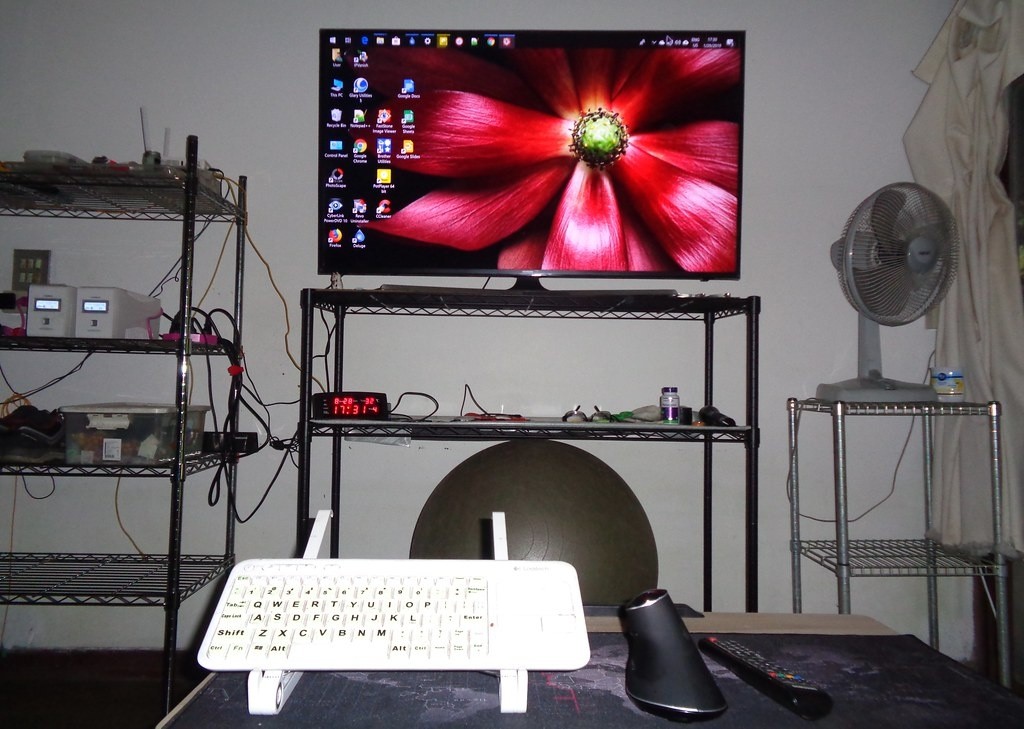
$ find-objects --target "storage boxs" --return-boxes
[60,401,211,464]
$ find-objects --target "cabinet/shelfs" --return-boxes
[786,397,1014,691]
[0,134,248,729]
[295,288,761,613]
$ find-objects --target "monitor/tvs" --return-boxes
[318,28,747,297]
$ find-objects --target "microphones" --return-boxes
[699,406,736,428]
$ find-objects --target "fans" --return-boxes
[816,181,961,402]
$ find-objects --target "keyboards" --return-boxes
[197,558,591,671]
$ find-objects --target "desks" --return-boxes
[153,612,1024,729]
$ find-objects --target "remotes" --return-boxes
[700,635,833,719]
